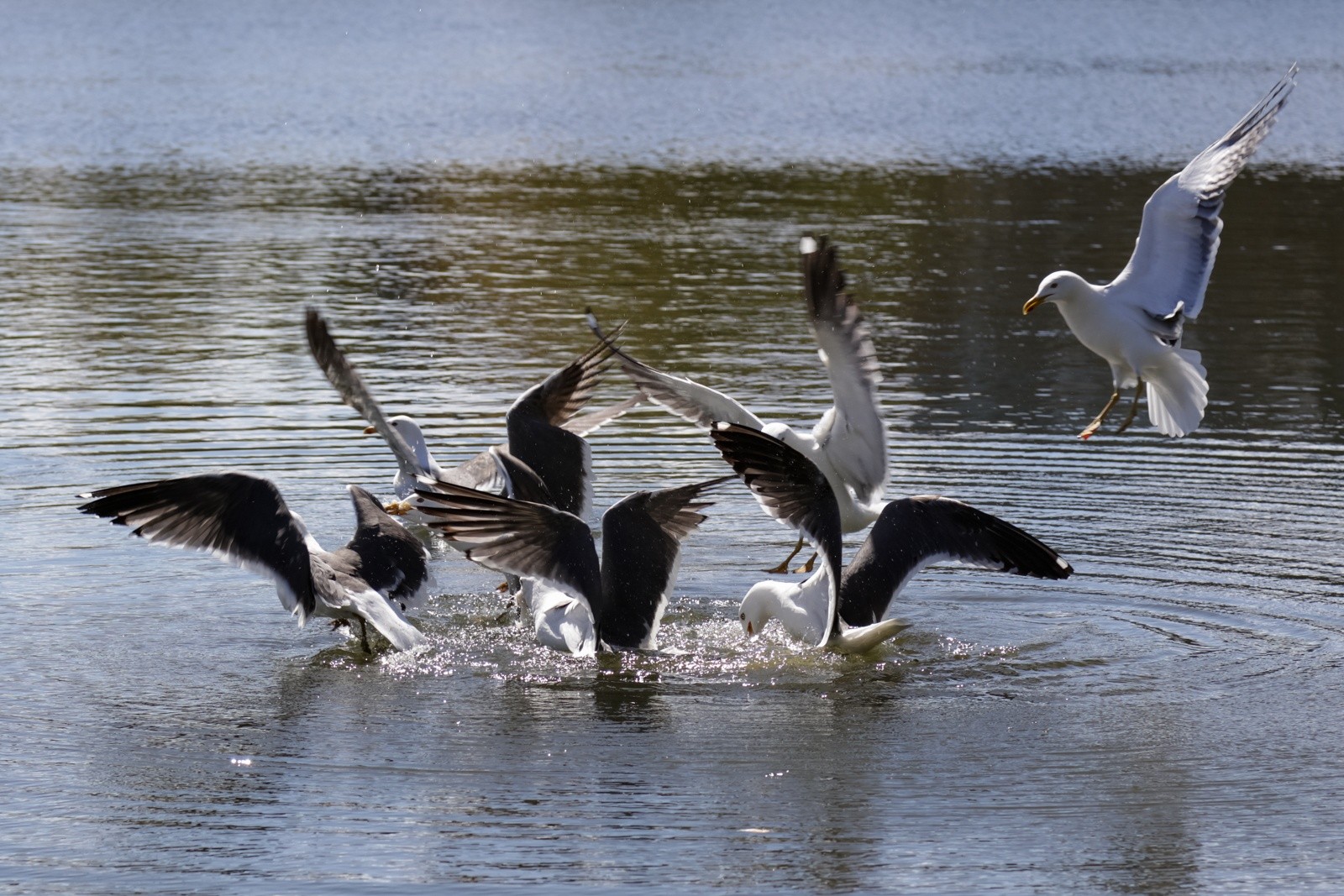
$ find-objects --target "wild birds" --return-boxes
[712,418,1073,660]
[584,223,889,575]
[410,472,742,649]
[1023,61,1301,439]
[65,307,646,650]
